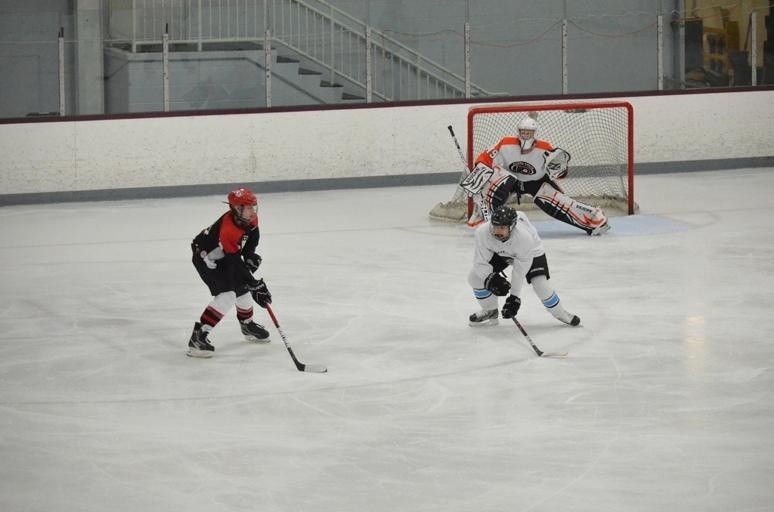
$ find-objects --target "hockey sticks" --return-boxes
[266,302,327,373]
[505,297,568,358]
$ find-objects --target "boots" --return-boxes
[237,314,269,339]
[189,322,214,351]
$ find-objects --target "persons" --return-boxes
[457,117,613,236]
[184,186,272,353]
[467,207,584,328]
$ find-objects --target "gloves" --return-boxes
[244,252,262,273]
[483,271,511,296]
[501,295,521,319]
[246,277,272,307]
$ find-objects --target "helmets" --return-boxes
[228,188,258,223]
[517,116,538,152]
[488,205,518,241]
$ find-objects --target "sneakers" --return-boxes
[470,309,498,322]
[556,308,580,326]
